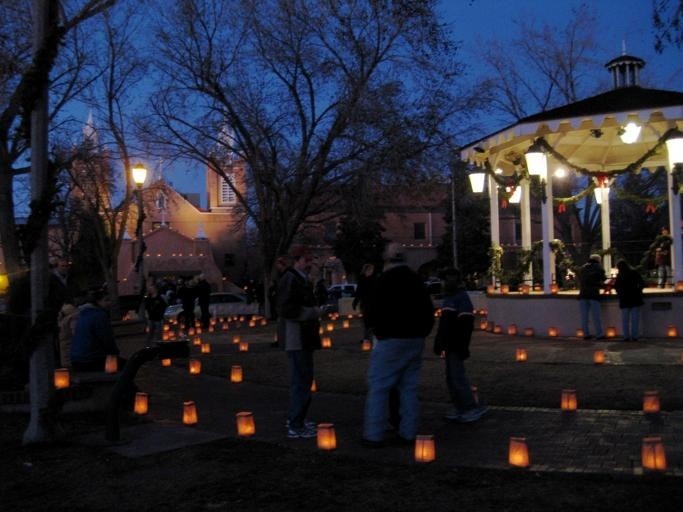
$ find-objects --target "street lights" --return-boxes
[445,157,459,270]
[130,160,147,320]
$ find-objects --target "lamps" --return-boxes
[465,167,488,193]
[524,137,547,175]
[658,127,683,165]
[593,177,610,204]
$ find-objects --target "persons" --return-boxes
[614,259,645,342]
[358,241,436,450]
[432,268,483,423]
[654,225,673,287]
[577,254,609,340]
[144,273,213,323]
[68,289,153,427]
[246,255,378,349]
[278,241,327,439]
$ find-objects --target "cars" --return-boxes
[327,284,357,296]
[163,292,260,321]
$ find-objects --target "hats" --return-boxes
[590,253,601,262]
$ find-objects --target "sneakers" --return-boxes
[286,427,318,439]
[458,404,488,424]
[285,420,316,429]
[444,408,461,421]
